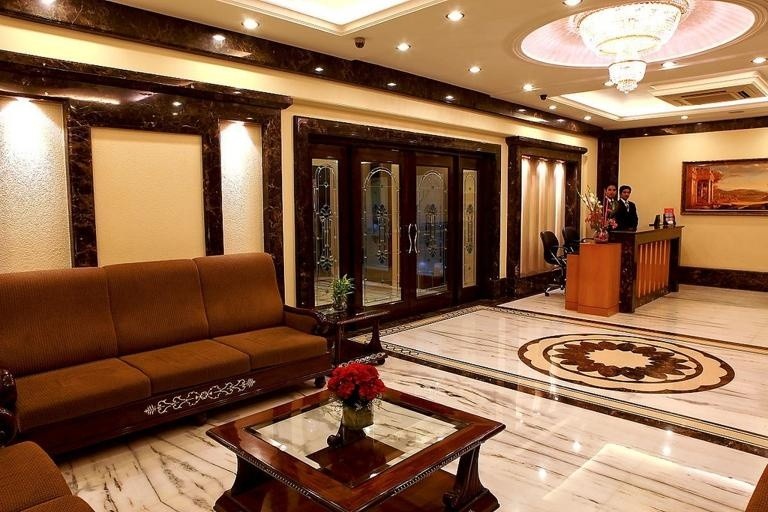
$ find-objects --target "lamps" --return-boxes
[573,0,690,96]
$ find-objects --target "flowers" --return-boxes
[576,184,618,229]
[320,362,388,402]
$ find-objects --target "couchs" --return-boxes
[0,252,336,454]
[0,442,96,512]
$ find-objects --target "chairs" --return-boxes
[540,230,568,296]
[562,227,593,257]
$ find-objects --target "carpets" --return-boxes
[343,300,767,461]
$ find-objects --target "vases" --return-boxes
[343,405,372,429]
[595,229,608,243]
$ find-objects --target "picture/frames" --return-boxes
[681,158,768,216]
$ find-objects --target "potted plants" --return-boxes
[326,273,355,310]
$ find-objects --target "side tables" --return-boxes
[312,304,390,365]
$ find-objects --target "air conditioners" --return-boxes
[656,84,766,107]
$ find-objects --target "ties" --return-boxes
[625,199,630,214]
[610,199,616,211]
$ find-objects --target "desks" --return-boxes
[564,224,685,317]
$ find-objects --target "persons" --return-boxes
[616,186,638,230]
[601,185,624,230]
